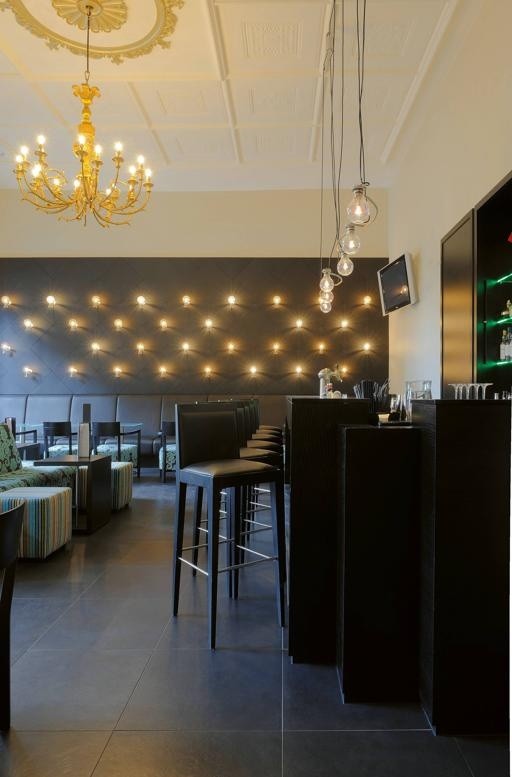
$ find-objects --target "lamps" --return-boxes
[0,0,185,225]
[318,35,334,313]
[341,2,360,256]
[336,33,355,277]
[347,0,379,227]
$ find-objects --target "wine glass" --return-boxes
[448,382,493,399]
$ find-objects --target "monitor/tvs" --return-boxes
[377,252,417,316]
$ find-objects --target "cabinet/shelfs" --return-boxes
[487,271,512,370]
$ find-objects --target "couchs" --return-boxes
[0,424,76,561]
[158,420,176,484]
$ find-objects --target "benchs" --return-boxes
[0,394,248,467]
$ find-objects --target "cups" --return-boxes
[318,367,329,396]
[326,383,332,396]
[403,380,432,419]
[390,394,402,419]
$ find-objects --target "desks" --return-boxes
[34,453,113,536]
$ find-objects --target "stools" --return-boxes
[110,461,134,513]
[0,486,72,561]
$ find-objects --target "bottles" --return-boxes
[499,326,512,361]
[493,388,511,400]
[500,299,511,316]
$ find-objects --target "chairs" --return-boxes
[172,394,284,655]
[1,502,26,734]
